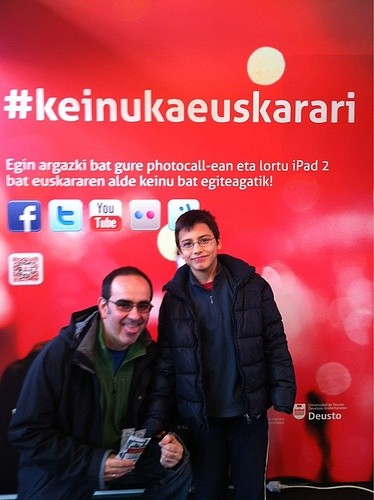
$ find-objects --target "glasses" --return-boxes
[180,237,215,248]
[107,298,154,313]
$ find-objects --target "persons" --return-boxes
[157,210,296,500]
[11,266,190,500]
